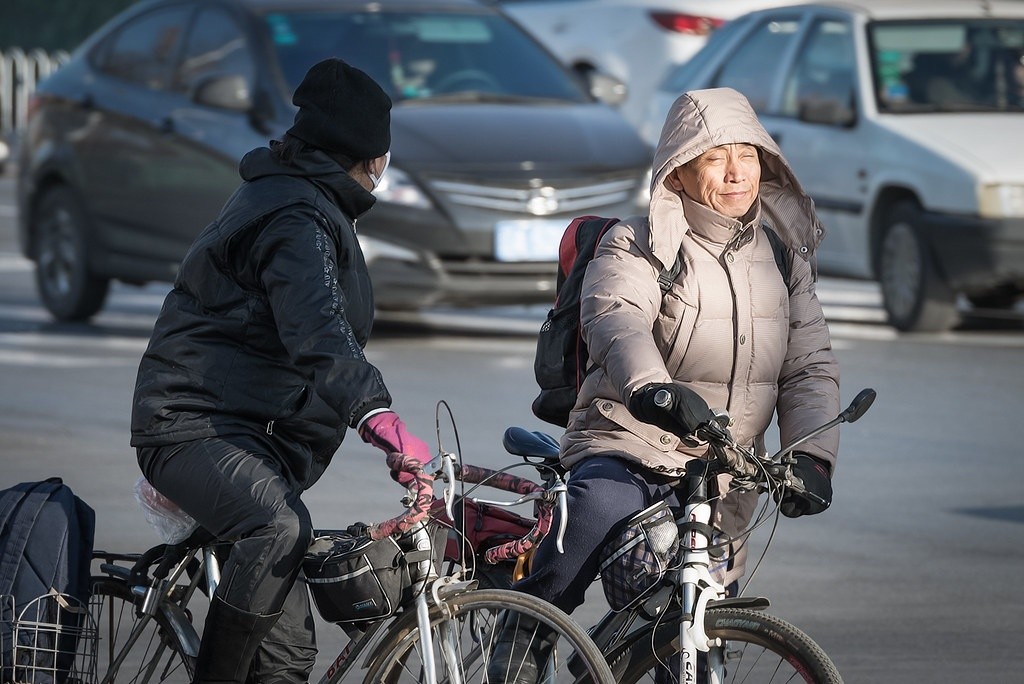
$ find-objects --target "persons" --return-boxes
[486,88,840,684]
[130,58,432,684]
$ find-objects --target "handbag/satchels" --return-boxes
[427,494,538,563]
[306,534,410,623]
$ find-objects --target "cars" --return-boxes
[647,2,1022,338]
[17,0,661,326]
[500,1,784,153]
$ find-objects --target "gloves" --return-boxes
[631,383,709,447]
[776,459,833,518]
[366,414,431,482]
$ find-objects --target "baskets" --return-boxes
[1,595,97,684]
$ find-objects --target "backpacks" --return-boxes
[531,215,678,429]
[0,478,102,683]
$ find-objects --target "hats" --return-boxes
[286,58,391,159]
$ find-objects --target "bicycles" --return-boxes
[420,386,878,684]
[67,475,616,684]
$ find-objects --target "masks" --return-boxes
[365,152,390,188]
